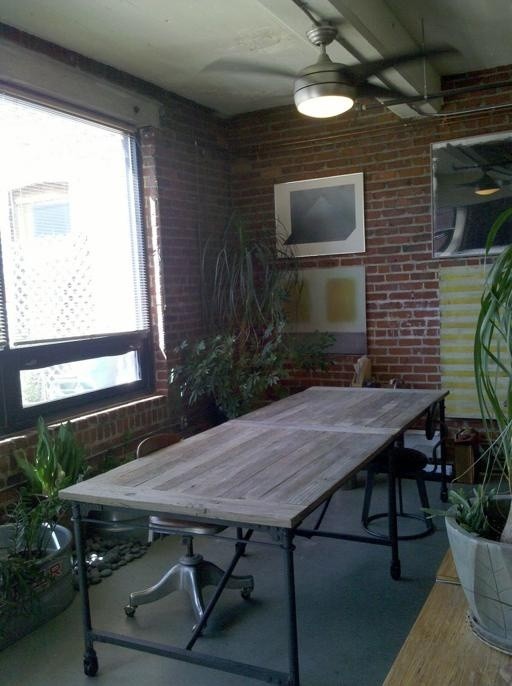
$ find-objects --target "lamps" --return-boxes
[293,83,356,118]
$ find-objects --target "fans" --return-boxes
[198,26,460,114]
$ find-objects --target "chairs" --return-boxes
[123,432,254,636]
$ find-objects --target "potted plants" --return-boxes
[0,555,33,649]
[0,473,88,622]
[89,428,160,539]
[443,207,512,656]
[7,417,87,523]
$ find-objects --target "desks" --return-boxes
[381,547,511,686]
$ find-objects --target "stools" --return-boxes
[360,447,435,540]
